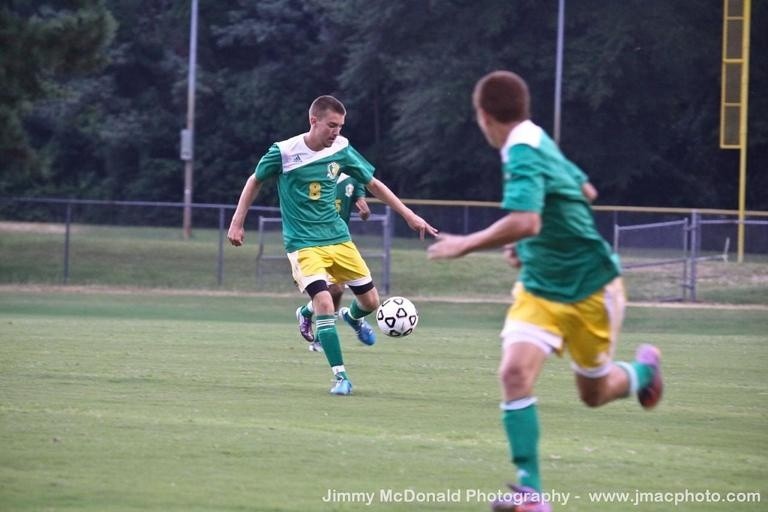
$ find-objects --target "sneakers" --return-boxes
[491,483,553,511]
[295,305,375,394]
[634,342,663,408]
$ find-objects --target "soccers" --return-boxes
[375,294,419,339]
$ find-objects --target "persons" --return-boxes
[295,171,372,354]
[428,70,666,512]
[226,94,439,396]
